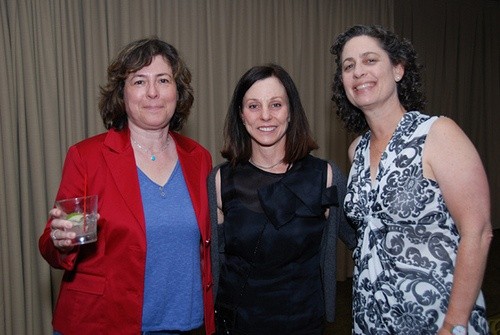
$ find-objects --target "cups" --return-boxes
[55,195,98,246]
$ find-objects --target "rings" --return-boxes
[52,230,58,240]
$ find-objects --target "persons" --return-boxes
[38,37,217,335]
[329,25,493,335]
[207,64,358,335]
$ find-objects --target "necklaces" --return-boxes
[134,137,170,161]
[251,159,283,169]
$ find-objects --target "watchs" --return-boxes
[443,321,468,335]
